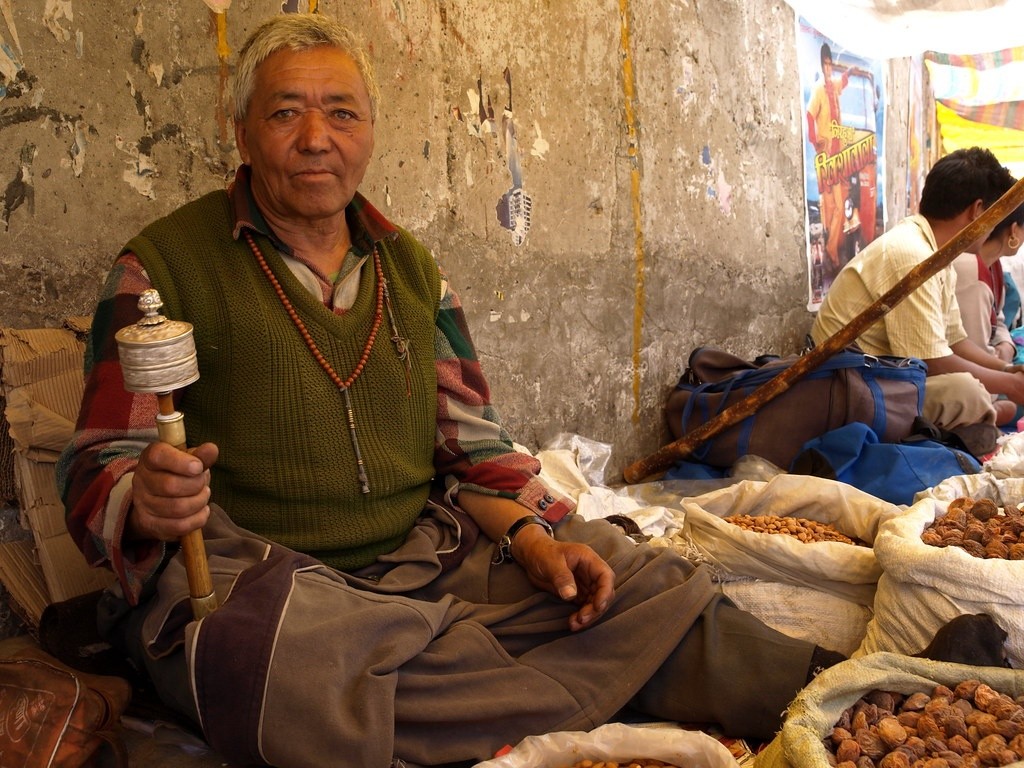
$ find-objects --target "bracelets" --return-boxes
[497,514,554,559]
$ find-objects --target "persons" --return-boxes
[806,42,859,271]
[951,176,1023,363]
[803,147,1024,454]
[53,13,1008,768]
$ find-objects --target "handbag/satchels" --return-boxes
[0,635,133,768]
[664,347,930,471]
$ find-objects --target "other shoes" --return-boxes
[941,419,998,453]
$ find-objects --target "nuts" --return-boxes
[719,513,854,546]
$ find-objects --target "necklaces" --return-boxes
[248,233,383,495]
